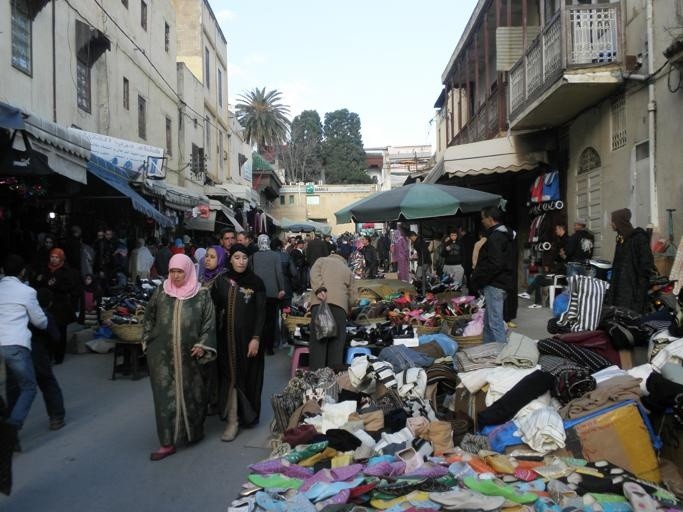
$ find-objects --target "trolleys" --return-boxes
[525,169,563,251]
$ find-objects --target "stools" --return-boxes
[543,273,566,310]
[111,339,146,380]
[343,345,372,366]
[290,346,310,380]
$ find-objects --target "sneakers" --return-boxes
[518,291,531,300]
[528,304,542,309]
[508,321,518,328]
[49,418,67,430]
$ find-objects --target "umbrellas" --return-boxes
[333,177,502,297]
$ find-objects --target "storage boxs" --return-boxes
[567,400,661,487]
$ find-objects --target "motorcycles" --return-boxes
[646,265,678,312]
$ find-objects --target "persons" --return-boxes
[609,208,651,313]
[472,206,519,343]
[0,206,470,496]
[518,217,594,308]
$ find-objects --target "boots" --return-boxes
[221,388,240,442]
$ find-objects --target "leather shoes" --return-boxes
[150,447,175,460]
